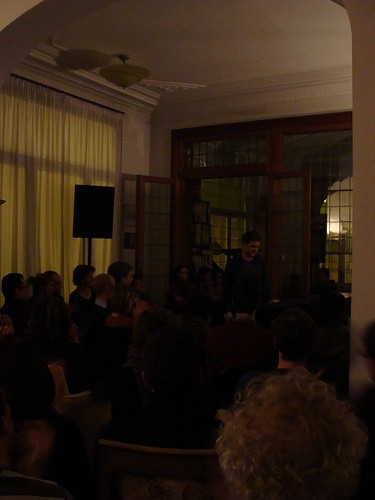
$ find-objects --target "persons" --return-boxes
[0,231,351,500]
[356,323,375,500]
[214,366,368,500]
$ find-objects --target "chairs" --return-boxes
[92,438,221,500]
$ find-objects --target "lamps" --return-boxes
[100,47,151,92]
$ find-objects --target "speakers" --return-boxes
[72,184,115,239]
